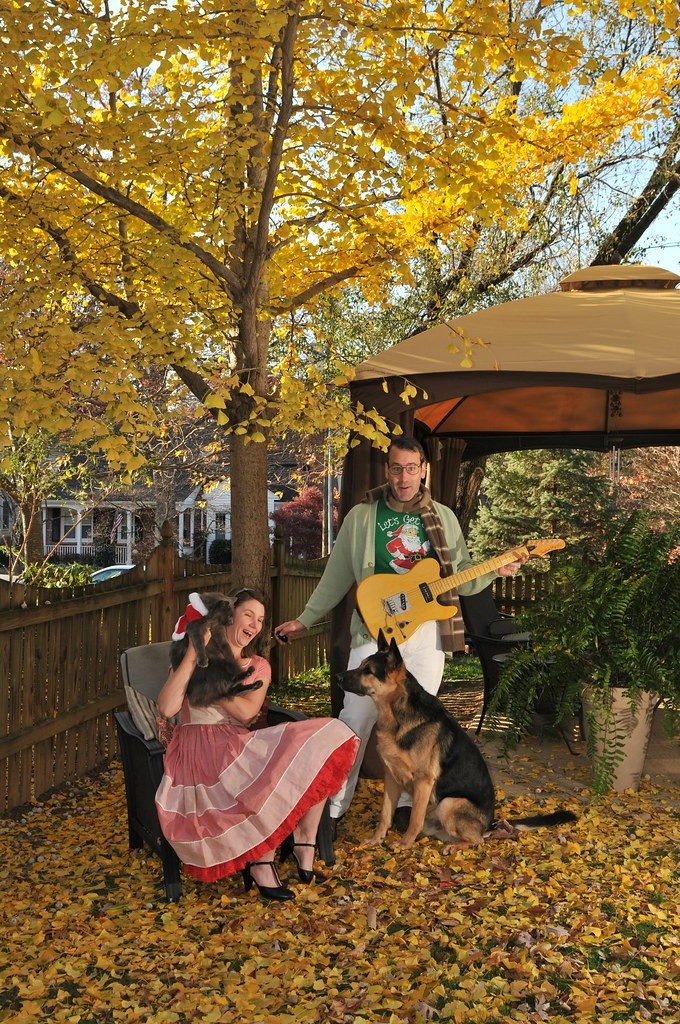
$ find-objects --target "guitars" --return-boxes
[355,536,567,649]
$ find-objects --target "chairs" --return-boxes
[460,583,587,756]
[113,639,335,903]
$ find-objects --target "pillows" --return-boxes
[124,686,180,740]
[156,716,177,748]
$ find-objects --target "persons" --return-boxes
[154,588,362,901]
[274,437,528,839]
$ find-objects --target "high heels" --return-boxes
[241,861,296,900]
[279,832,327,884]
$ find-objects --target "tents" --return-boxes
[326,265,680,782]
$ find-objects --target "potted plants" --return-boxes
[477,508,679,798]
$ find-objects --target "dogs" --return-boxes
[331,628,578,850]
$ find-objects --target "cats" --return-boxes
[169,592,263,709]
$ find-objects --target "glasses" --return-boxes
[388,463,422,475]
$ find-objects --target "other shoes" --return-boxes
[396,806,412,832]
[330,813,345,841]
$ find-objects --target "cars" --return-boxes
[85,564,141,585]
